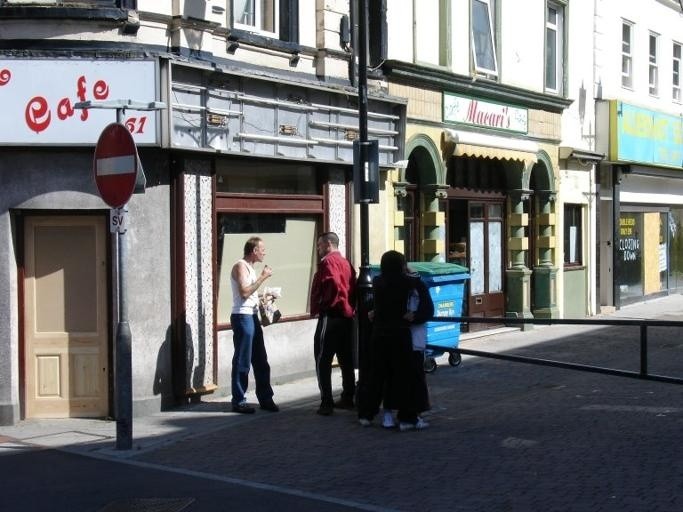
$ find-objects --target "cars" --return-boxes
[593,231,664,289]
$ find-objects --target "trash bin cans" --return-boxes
[368,261,471,373]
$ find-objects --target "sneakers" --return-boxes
[259,403,280,414]
[231,404,255,414]
[316,407,334,417]
[359,413,433,429]
[335,400,354,411]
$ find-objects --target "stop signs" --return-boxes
[90,126,139,212]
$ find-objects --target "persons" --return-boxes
[356,251,421,432]
[368,254,435,429]
[310,232,355,416]
[230,238,279,414]
